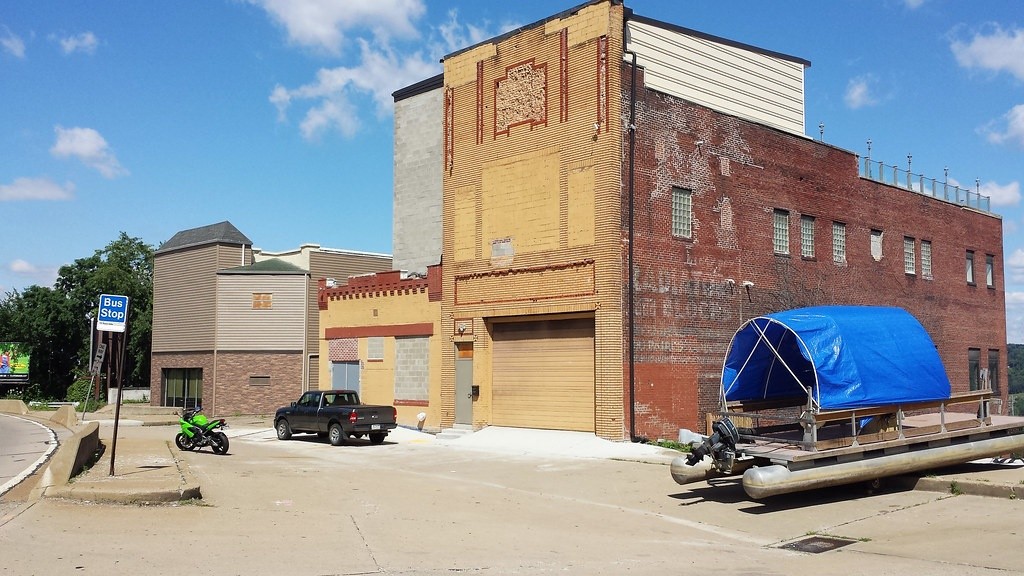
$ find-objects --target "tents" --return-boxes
[719,306,950,417]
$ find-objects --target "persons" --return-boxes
[0,351,11,373]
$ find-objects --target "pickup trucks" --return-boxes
[274,390,398,446]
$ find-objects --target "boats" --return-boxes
[671,306,1024,499]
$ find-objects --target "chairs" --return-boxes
[331,396,348,405]
[316,398,330,406]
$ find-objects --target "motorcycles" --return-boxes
[172,408,229,455]
